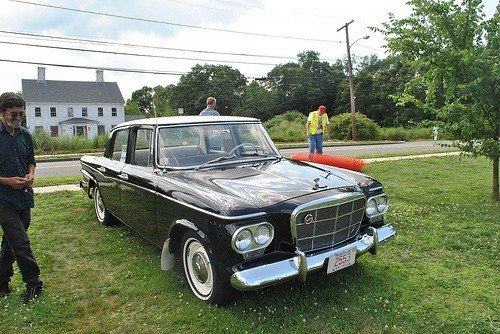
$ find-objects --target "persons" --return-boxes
[0,92,44,304]
[306,106,329,162]
[200,97,222,151]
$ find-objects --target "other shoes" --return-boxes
[23,286,42,303]
[0,288,10,301]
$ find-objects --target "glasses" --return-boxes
[8,111,27,117]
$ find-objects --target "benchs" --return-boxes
[134,145,224,166]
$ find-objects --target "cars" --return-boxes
[80,115,397,308]
